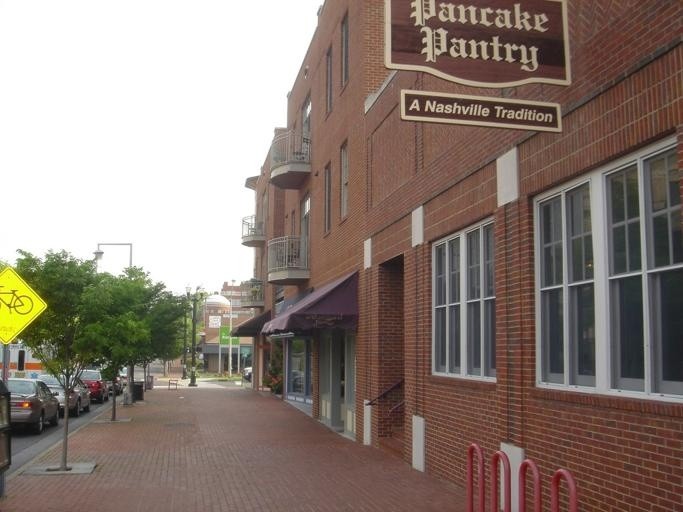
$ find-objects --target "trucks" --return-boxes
[0,343,58,377]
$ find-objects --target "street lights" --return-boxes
[92,242,134,402]
[228,278,235,376]
[180,305,193,380]
[184,283,205,386]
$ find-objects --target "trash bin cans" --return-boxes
[132,381,143,401]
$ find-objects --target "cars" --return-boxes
[118,366,127,385]
[74,369,109,402]
[292,374,304,392]
[5,377,60,432]
[95,367,123,395]
[291,370,300,378]
[37,375,91,417]
[242,367,251,381]
